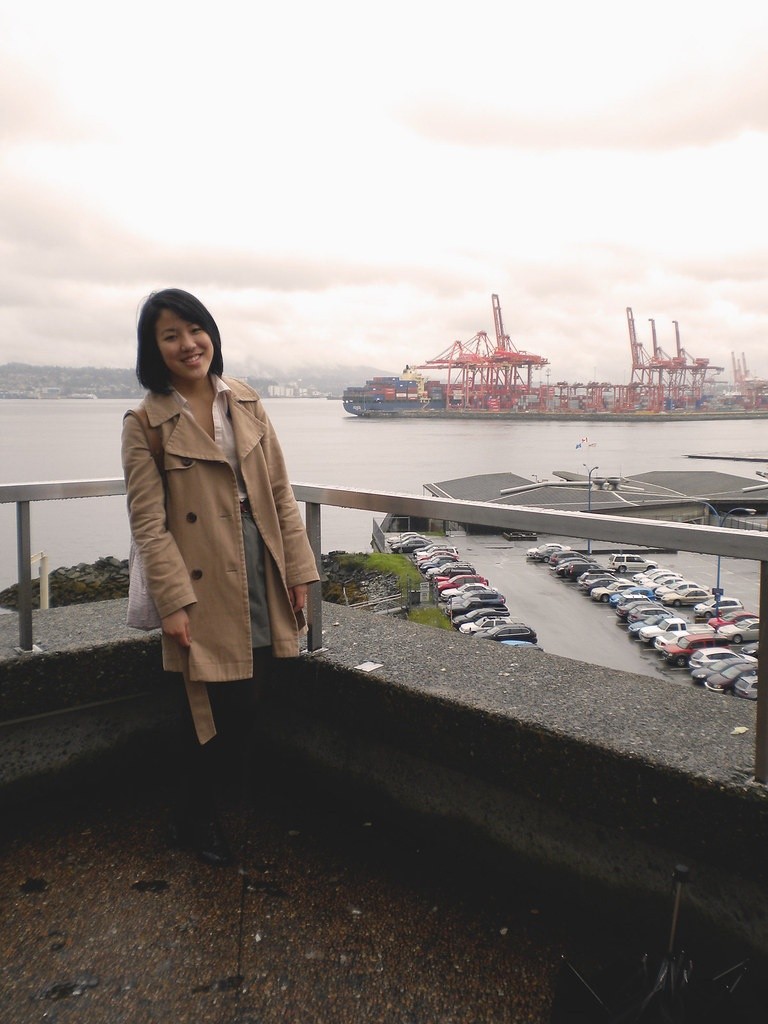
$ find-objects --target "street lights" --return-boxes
[693,498,756,633]
[583,463,599,554]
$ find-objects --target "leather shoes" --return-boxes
[193,817,230,866]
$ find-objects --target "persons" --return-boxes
[121,289,321,867]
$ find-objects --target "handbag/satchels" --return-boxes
[126,535,162,630]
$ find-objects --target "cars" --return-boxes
[387,532,543,651]
[527,542,759,700]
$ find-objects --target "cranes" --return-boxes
[403,294,768,413]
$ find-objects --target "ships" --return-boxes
[342,377,526,415]
